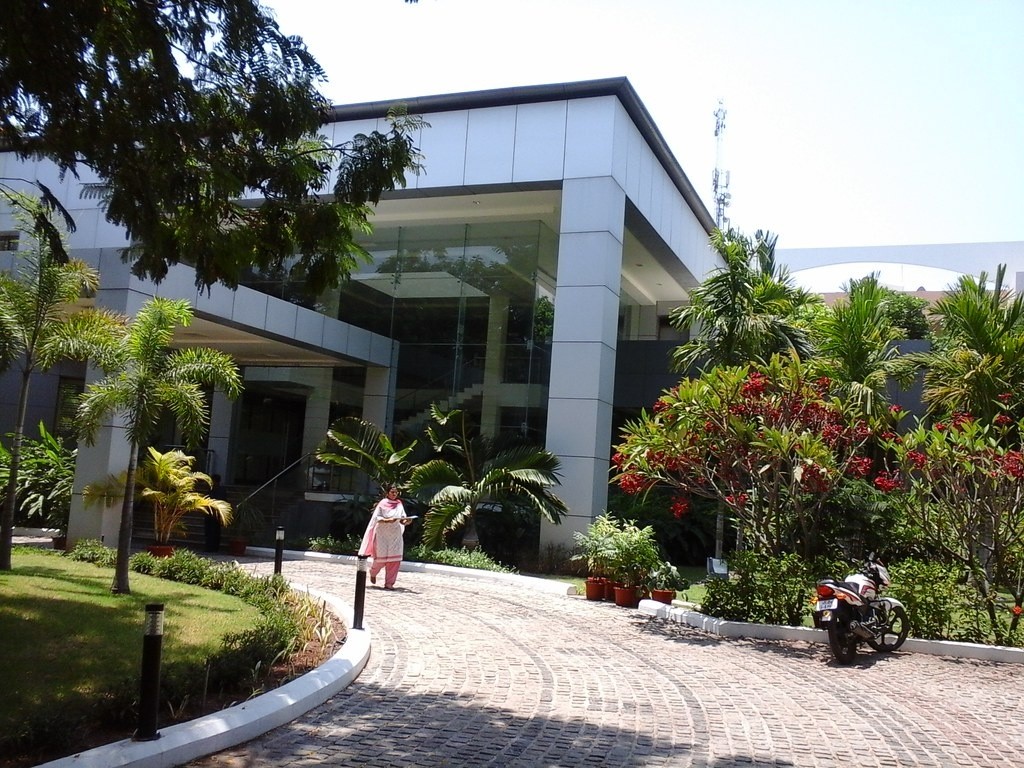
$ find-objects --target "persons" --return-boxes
[358,485,412,591]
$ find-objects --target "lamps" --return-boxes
[276,526,284,540]
[144,604,164,635]
[357,554,368,571]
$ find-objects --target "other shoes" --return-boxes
[370,570,377,584]
[384,585,396,591]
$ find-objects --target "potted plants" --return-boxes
[570,509,690,608]
[83,447,234,558]
[47,494,71,550]
[402,400,569,563]
[316,415,430,546]
[226,492,264,554]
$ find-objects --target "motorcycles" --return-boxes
[810,551,910,666]
